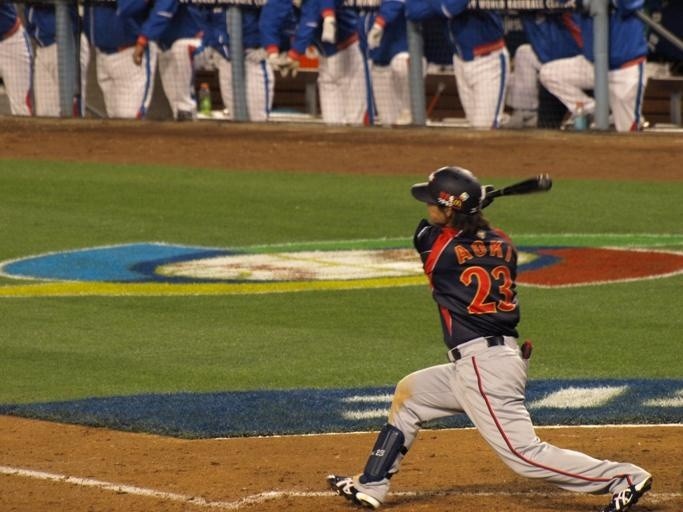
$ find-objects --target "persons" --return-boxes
[323,165,656,511]
[0,1,652,133]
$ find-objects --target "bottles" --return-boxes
[197,84,212,116]
[573,100,587,130]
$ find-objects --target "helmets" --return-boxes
[410,166,481,215]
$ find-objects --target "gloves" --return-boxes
[245,48,300,78]
[366,22,384,51]
[320,15,336,46]
[479,184,494,210]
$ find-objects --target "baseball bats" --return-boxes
[489,174,552,195]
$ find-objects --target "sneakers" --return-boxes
[602,474,652,511]
[561,104,612,131]
[504,110,539,128]
[326,473,390,510]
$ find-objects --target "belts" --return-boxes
[451,335,504,360]
